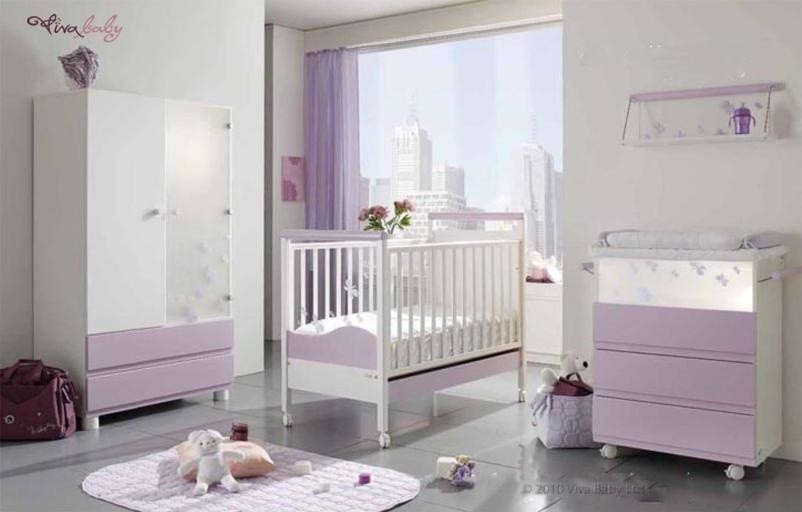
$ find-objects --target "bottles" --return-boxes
[728,103,756,134]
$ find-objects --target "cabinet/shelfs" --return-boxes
[27,89,236,433]
[586,243,784,482]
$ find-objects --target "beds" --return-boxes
[281,205,526,448]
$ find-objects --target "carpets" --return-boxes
[79,436,422,512]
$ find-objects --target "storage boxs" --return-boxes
[530,370,600,450]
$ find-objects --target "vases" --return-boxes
[380,232,398,240]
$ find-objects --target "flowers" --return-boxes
[358,199,415,240]
